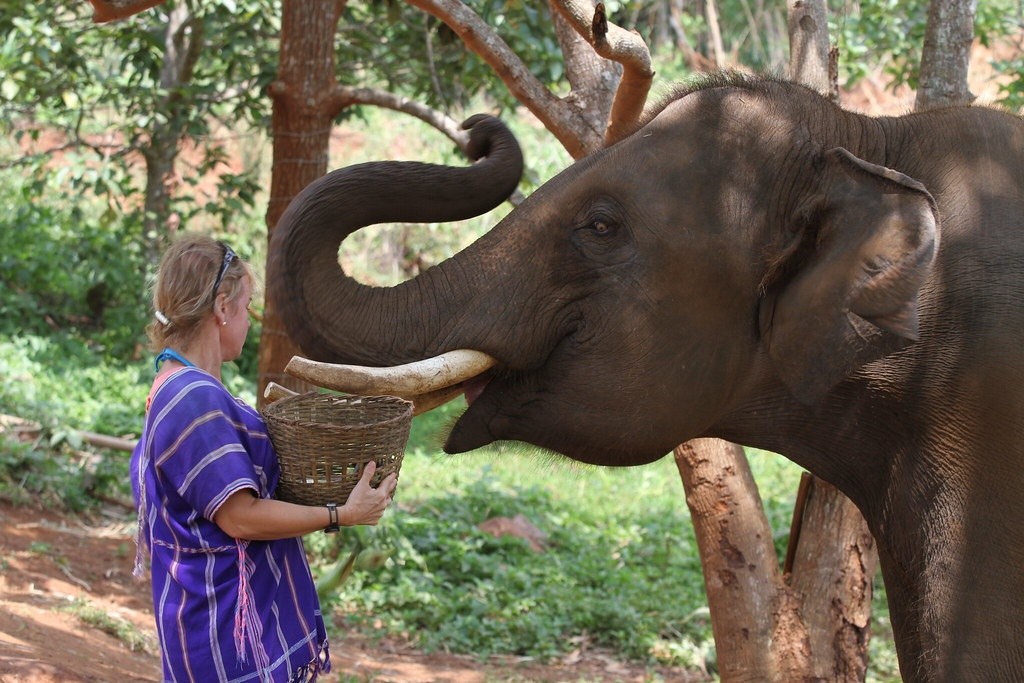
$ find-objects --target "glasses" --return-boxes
[212,240,239,301]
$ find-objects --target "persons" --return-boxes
[128,235,398,683]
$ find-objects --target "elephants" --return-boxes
[268,69,1023,683]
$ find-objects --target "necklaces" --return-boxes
[155,348,195,372]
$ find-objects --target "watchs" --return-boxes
[324,504,340,533]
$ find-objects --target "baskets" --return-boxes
[260,393,415,507]
